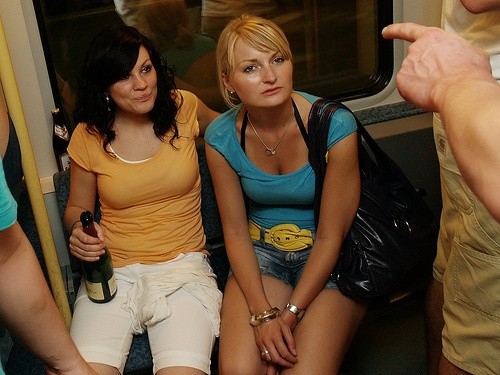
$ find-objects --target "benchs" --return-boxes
[52,99,442,374]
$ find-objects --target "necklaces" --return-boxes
[246,109,295,156]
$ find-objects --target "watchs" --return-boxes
[285,303,305,321]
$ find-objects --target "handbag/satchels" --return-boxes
[307,99,443,316]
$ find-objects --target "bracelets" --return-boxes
[249,307,281,327]
[261,350,268,357]
[70,220,80,234]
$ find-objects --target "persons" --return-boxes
[205,14,371,375]
[383,22,500,225]
[114,0,374,108]
[435,0,500,375]
[65,26,222,375]
[0,153,99,375]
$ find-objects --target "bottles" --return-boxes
[51,107,72,172]
[80,210,118,303]
